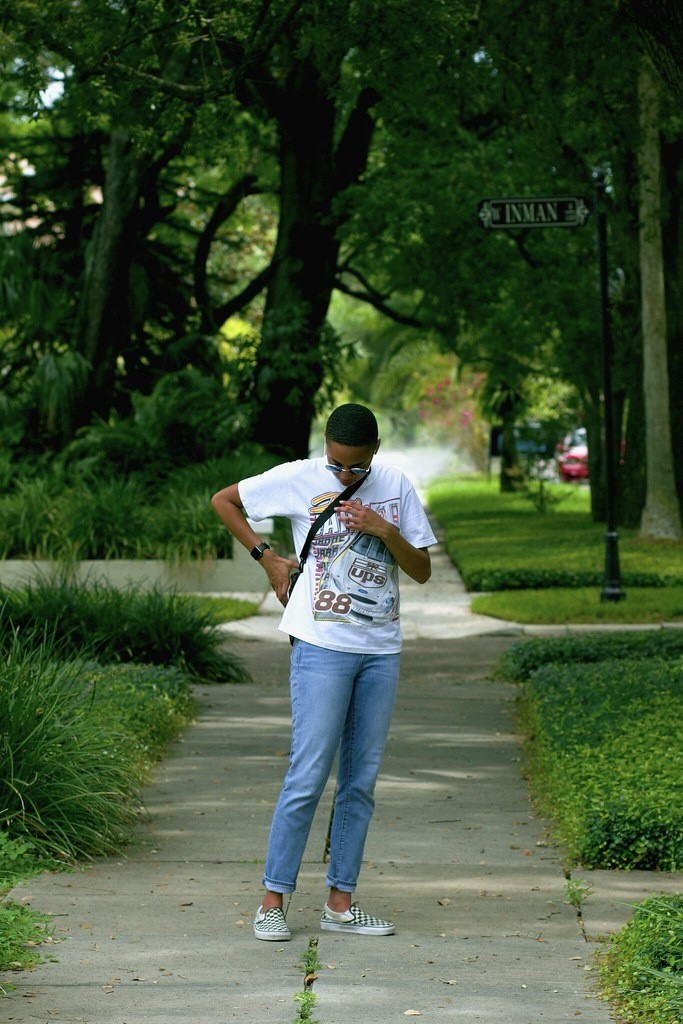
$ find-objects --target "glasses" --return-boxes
[323,437,377,475]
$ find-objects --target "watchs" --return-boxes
[251,543,270,561]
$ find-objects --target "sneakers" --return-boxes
[320,903,396,935]
[253,905,291,940]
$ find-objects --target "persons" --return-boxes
[211,404,438,940]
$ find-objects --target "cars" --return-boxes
[559,443,588,481]
